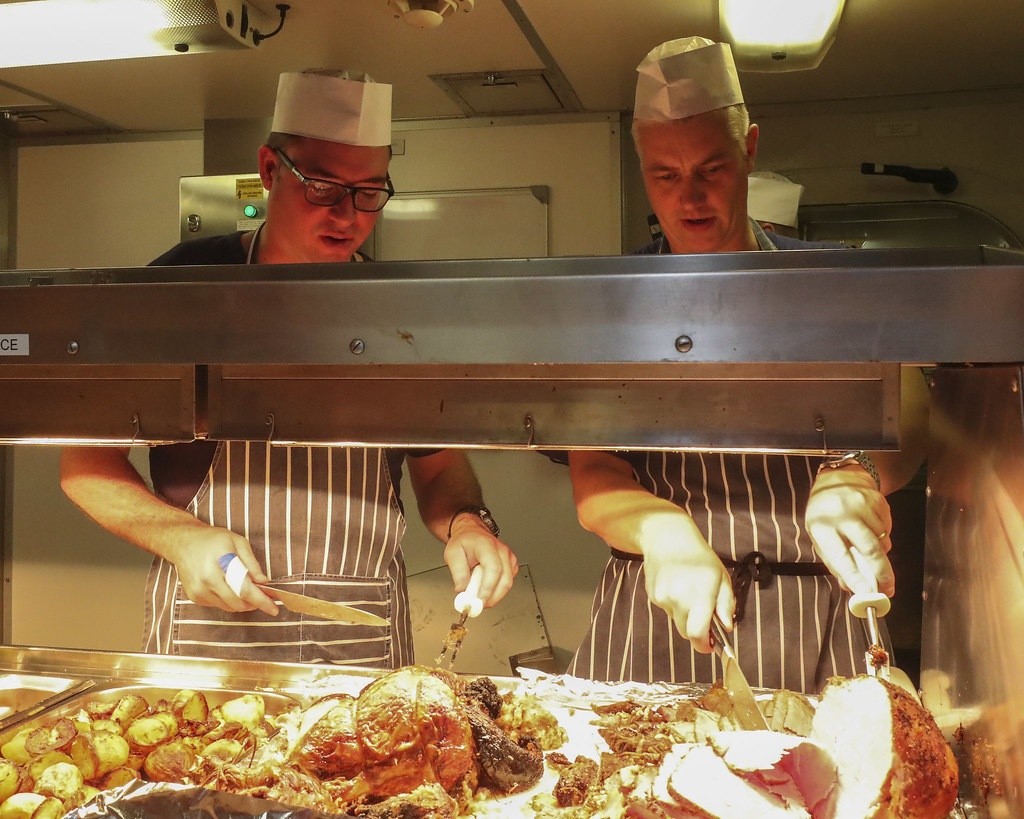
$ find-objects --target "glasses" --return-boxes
[268,145,395,213]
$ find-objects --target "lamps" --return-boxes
[719,0,847,73]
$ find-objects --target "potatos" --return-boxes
[0,687,265,819]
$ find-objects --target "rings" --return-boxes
[879,530,888,540]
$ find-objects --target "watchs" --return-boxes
[817,450,881,491]
[447,507,502,543]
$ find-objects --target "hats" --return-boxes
[272,68,392,146]
[633,36,744,120]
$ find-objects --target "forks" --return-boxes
[435,562,485,671]
[847,544,895,683]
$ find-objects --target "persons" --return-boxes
[533,35,937,688]
[56,67,524,676]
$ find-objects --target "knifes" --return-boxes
[702,610,771,734]
[249,579,390,628]
